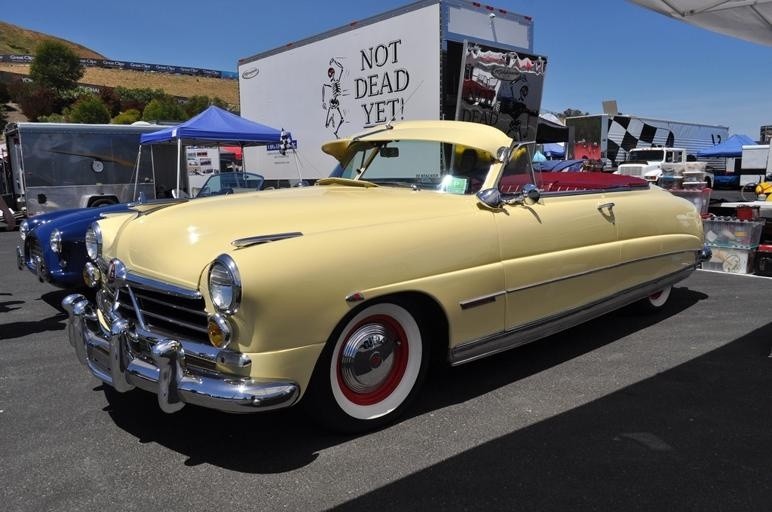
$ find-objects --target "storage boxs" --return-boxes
[702,217,766,273]
[659,161,711,219]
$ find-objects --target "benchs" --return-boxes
[500,171,649,194]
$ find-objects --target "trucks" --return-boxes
[3,122,236,218]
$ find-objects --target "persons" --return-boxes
[580,155,594,172]
[460,148,482,184]
[755,173,772,202]
